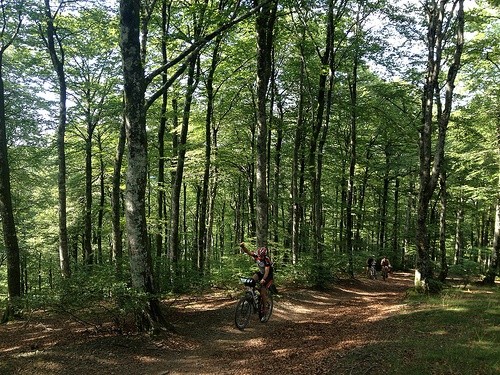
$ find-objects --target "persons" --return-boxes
[367,255,377,279]
[381,256,391,277]
[240,242,273,314]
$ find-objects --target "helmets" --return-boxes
[259,247,267,255]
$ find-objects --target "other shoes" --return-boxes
[260,310,267,316]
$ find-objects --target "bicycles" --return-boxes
[379,264,389,281]
[234,274,274,330]
[366,264,378,280]
